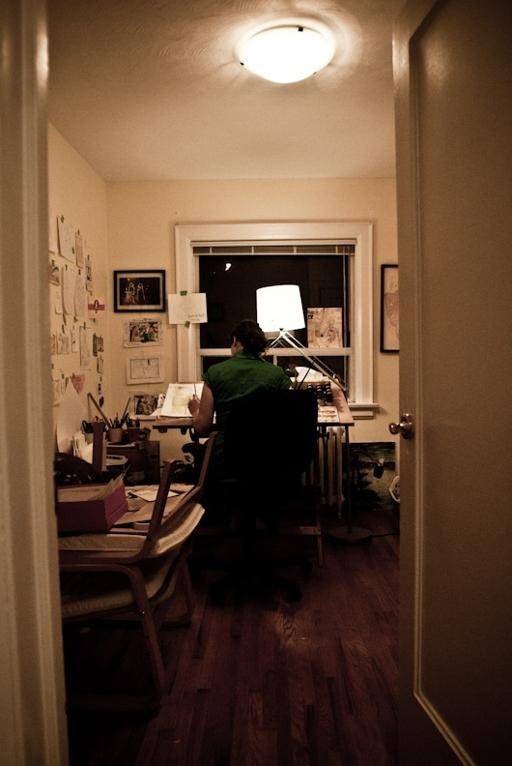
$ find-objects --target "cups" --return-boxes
[127,427,140,442]
[108,427,121,442]
[80,420,93,433]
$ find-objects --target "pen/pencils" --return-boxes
[109,396,140,429]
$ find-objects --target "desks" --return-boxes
[150,380,354,537]
[98,438,162,480]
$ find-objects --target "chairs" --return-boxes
[199,384,327,575]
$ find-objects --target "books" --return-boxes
[159,383,218,418]
[71,430,94,464]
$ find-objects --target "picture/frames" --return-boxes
[379,261,401,358]
[124,353,167,388]
[119,315,166,349]
[110,266,167,314]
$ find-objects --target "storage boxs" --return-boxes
[55,477,130,538]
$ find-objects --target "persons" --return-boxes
[188,321,295,481]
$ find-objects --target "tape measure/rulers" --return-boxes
[88,392,112,428]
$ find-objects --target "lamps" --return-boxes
[229,13,337,87]
[253,279,350,399]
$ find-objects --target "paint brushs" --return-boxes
[193,394,196,400]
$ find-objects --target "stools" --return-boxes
[56,550,201,707]
[51,428,221,562]
